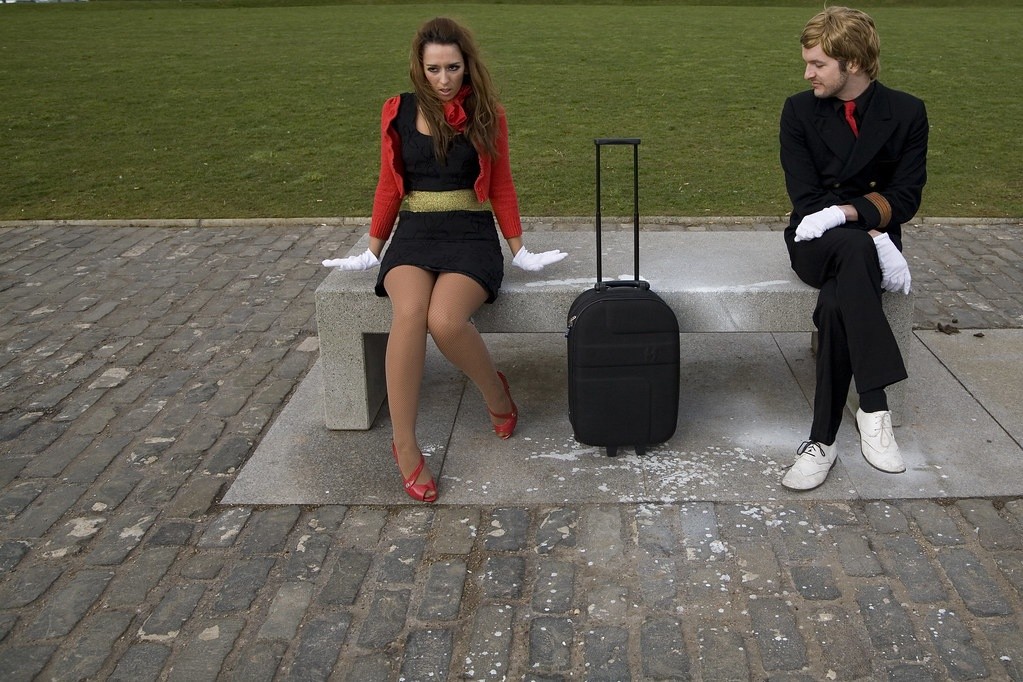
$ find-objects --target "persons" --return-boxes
[778,4,930,491]
[322,16,568,502]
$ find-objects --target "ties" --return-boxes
[845,101,860,140]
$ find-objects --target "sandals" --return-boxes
[394,442,437,502]
[486,372,518,438]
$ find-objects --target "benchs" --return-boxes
[315,232,914,431]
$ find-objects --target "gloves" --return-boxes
[794,205,847,242]
[873,232,912,294]
[511,245,568,272]
[321,248,380,272]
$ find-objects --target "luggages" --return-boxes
[564,137,682,456]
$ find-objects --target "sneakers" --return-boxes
[781,436,838,492]
[855,406,906,473]
